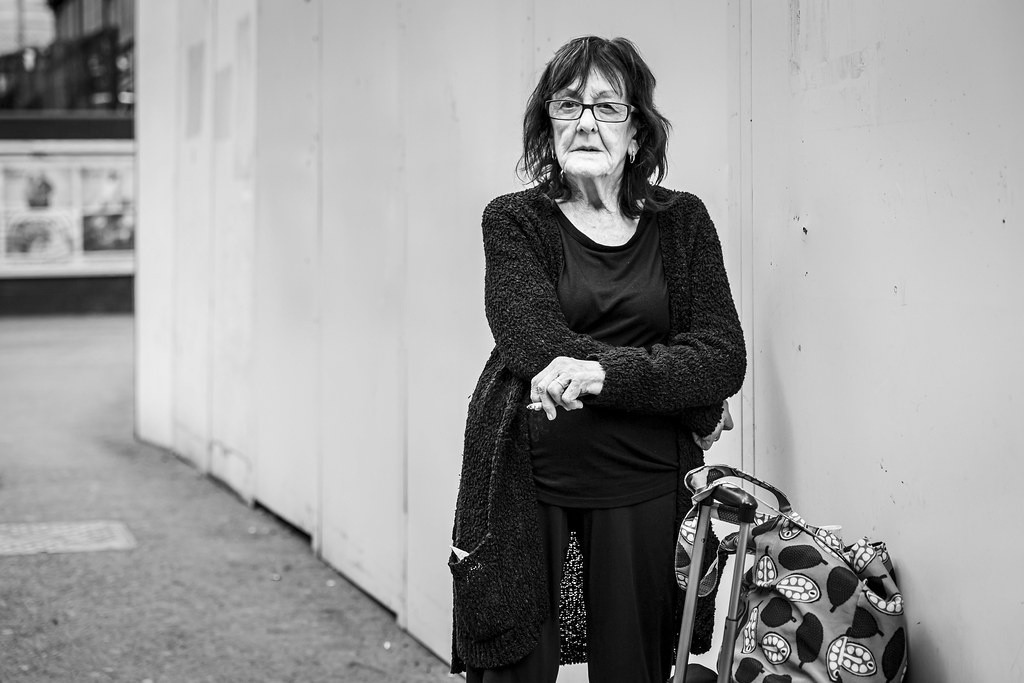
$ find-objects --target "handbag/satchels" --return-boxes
[673,464,909,683]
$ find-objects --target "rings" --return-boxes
[553,376,568,389]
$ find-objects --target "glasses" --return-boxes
[545,99,635,123]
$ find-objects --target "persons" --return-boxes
[446,33,747,683]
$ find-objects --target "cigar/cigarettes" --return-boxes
[526,402,543,411]
